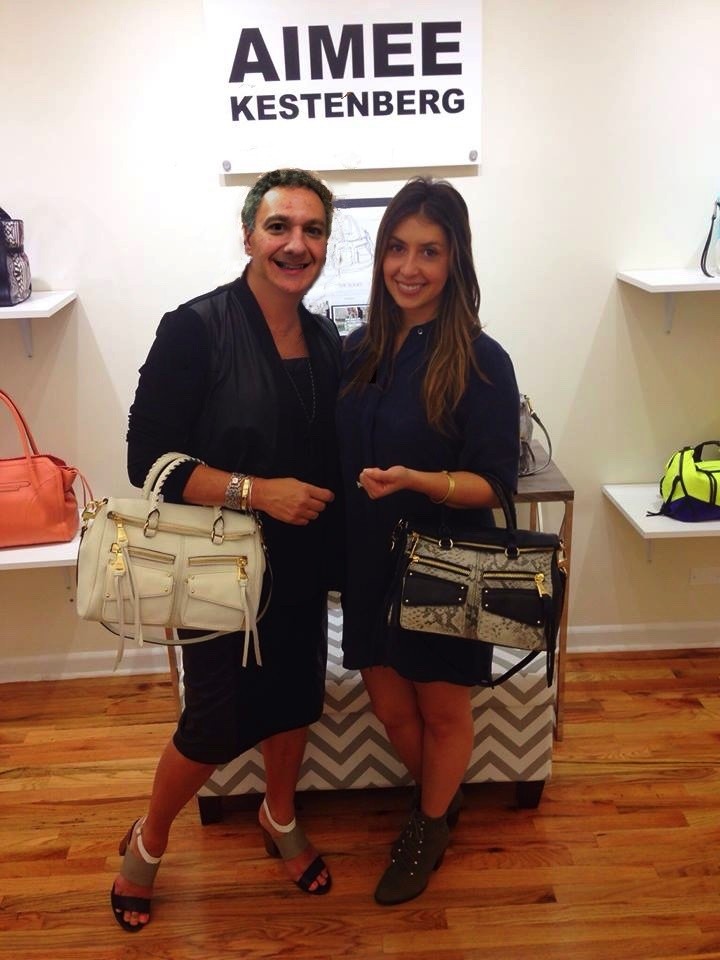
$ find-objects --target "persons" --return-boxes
[335,177,520,908]
[110,168,341,932]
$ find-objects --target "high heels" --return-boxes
[262,798,332,895]
[391,785,463,856]
[374,807,450,905]
[110,815,163,933]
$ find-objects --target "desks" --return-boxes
[512,440,574,744]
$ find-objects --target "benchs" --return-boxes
[176,592,556,827]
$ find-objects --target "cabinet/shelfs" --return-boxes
[601,271,720,537]
[0,292,80,589]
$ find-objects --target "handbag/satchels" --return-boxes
[0,207,33,307]
[646,440,720,522]
[517,392,552,478]
[0,388,94,548]
[76,452,273,673]
[375,468,568,689]
[700,195,720,278]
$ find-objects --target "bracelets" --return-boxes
[223,472,257,514]
[429,470,455,506]
